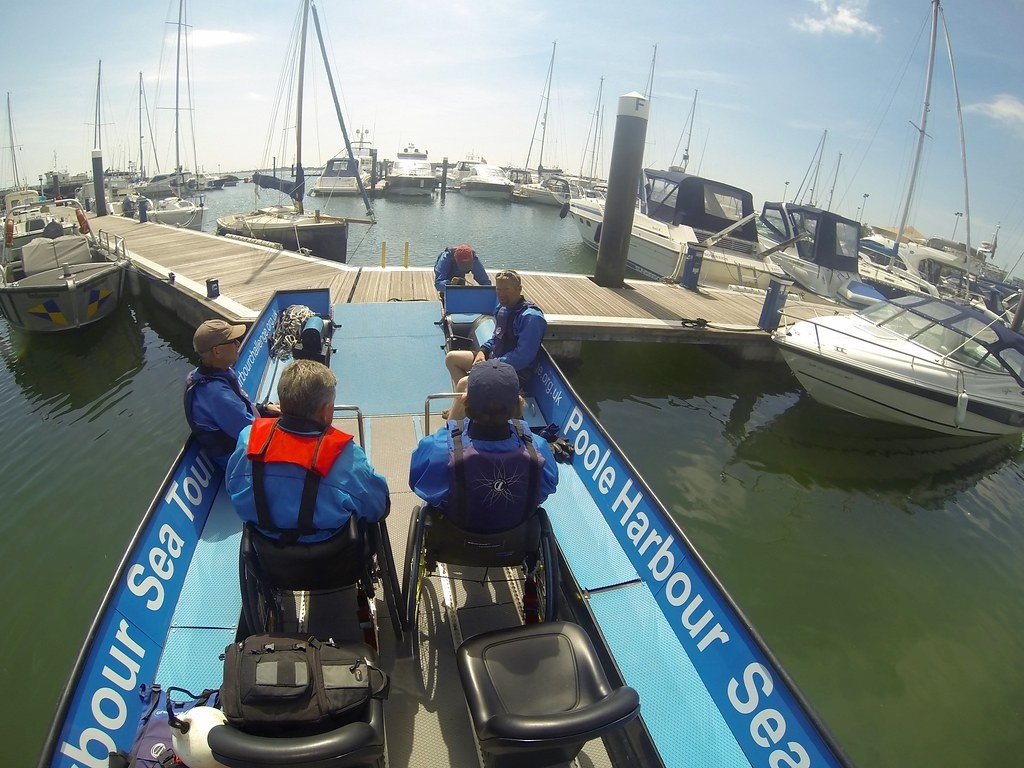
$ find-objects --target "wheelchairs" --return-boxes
[239,519,404,642]
[402,501,558,632]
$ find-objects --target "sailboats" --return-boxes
[216,0,377,263]
[133,0,209,231]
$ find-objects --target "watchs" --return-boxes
[264,401,273,413]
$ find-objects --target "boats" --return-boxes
[386,143,436,195]
[344,128,373,181]
[858,0,1024,329]
[0,199,131,333]
[134,173,239,197]
[0,91,53,243]
[569,164,786,294]
[459,164,516,197]
[313,158,371,195]
[446,158,481,188]
[75,60,140,218]
[508,40,711,202]
[758,200,861,297]
[519,177,586,207]
[771,286,1024,436]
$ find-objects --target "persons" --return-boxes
[409,359,559,535]
[442,270,547,421]
[433,245,491,309]
[224,359,390,542]
[184,319,280,473]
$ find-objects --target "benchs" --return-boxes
[448,313,751,768]
[148,353,297,719]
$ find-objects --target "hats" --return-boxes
[468,361,519,415]
[454,244,473,263]
[192,319,246,352]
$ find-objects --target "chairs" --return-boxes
[454,620,640,768]
[206,629,385,768]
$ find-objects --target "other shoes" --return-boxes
[442,408,450,419]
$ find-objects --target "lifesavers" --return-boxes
[538,165,544,176]
[5,219,14,248]
[75,207,90,234]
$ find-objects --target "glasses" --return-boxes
[496,272,519,282]
[219,339,237,345]
